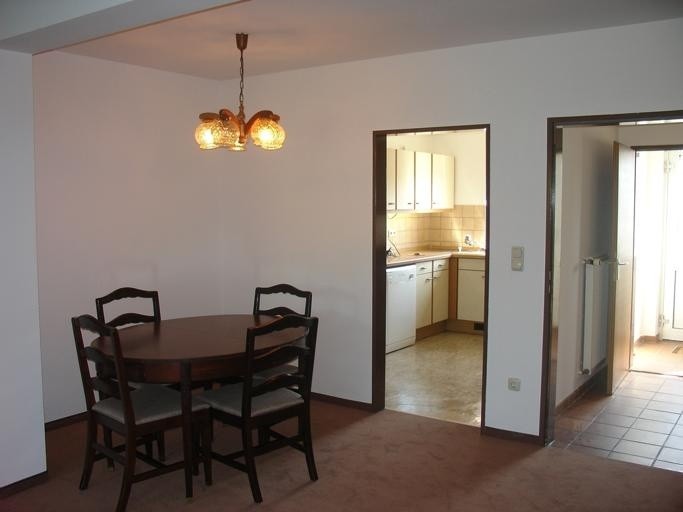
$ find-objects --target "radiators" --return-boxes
[581,258,610,379]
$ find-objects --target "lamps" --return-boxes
[194,33,286,153]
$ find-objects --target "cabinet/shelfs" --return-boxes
[456,257,486,325]
[416,258,450,330]
[386,263,416,356]
[386,149,455,211]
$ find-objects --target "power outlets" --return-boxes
[508,377,521,390]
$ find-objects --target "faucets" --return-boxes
[464,235,473,246]
